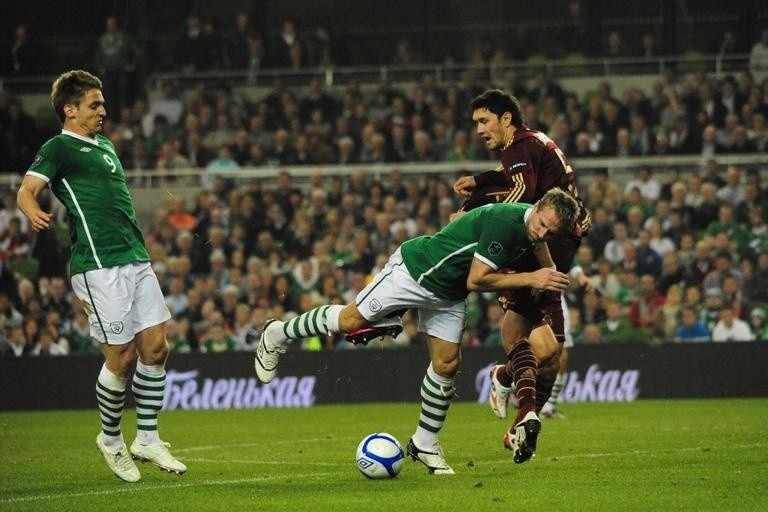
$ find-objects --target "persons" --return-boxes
[2,1,768,356]
[256,187,579,475]
[449,88,591,458]
[17,69,188,481]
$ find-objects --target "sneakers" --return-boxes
[489,364,564,418]
[129,437,188,475]
[405,434,456,475]
[503,411,541,463]
[95,431,141,483]
[345,315,404,346]
[254,318,287,384]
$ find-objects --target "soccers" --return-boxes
[357,432,404,480]
